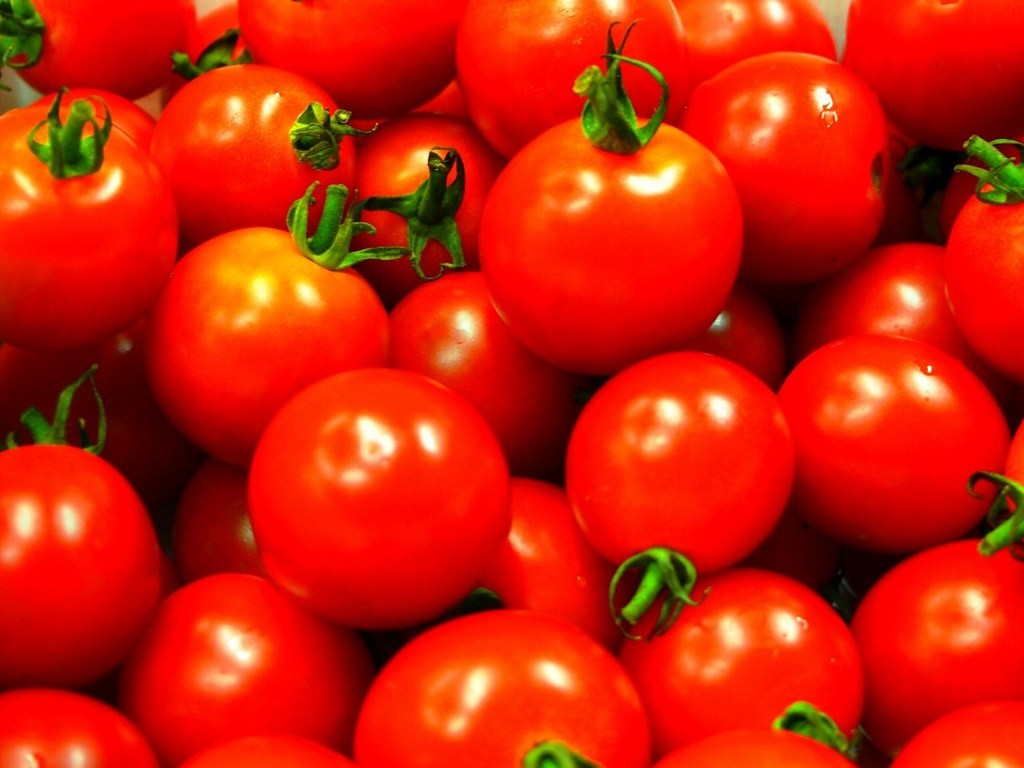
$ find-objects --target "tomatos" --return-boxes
[0,0,1024,768]
